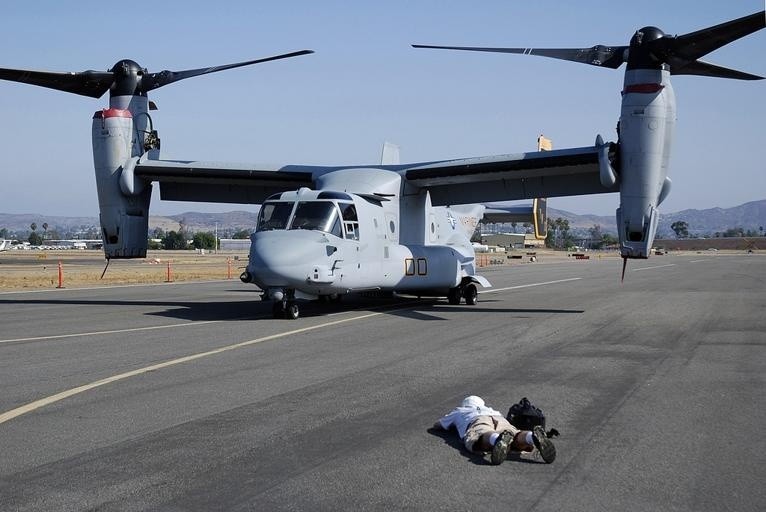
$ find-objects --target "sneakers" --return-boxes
[532,426,557,464]
[491,429,515,465]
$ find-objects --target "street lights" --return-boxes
[213,219,218,253]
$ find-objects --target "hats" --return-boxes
[461,396,485,407]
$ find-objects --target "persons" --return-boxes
[433,395,556,466]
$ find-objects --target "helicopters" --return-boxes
[0,12,766,320]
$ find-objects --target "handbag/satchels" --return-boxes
[506,396,545,431]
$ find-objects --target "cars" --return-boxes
[707,247,717,251]
[655,250,665,256]
[0,239,88,252]
[471,242,490,254]
